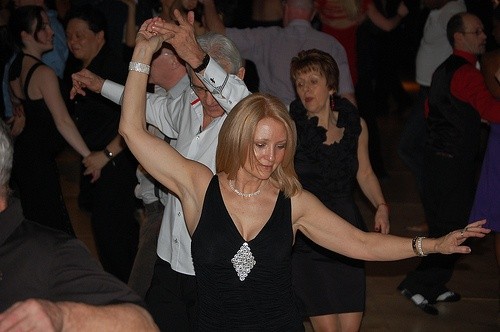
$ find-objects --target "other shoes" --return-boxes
[436,290,461,302]
[398,279,439,316]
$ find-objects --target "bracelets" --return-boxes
[128,62,151,75]
[376,203,388,210]
[412,236,427,257]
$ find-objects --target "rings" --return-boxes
[459,231,464,236]
[152,31,157,35]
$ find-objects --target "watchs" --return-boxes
[192,53,210,73]
[103,149,113,160]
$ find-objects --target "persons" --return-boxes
[2,5,78,249]
[116,16,490,332]
[202,0,355,111]
[0,129,163,332]
[397,12,499,315]
[60,13,141,286]
[315,1,399,87]
[72,43,189,300]
[277,48,389,332]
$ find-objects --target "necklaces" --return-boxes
[229,179,265,198]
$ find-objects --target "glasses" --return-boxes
[460,27,486,36]
[190,82,210,93]
